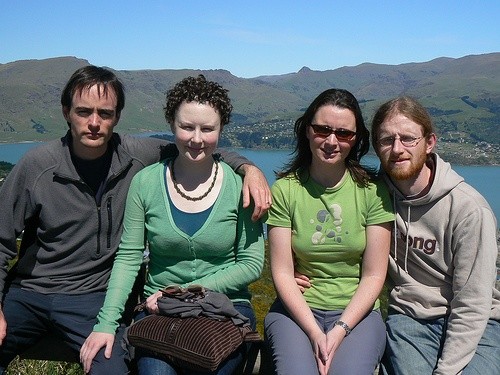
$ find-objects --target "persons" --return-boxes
[371,97,500,374]
[0,66,272,375]
[80,76,264,375]
[264,88,395,375]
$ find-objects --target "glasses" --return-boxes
[310,123,358,142]
[376,131,431,147]
[158,285,206,300]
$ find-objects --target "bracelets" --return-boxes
[333,320,351,337]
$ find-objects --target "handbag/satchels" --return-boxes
[126,312,249,370]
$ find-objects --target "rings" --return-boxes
[265,200,269,203]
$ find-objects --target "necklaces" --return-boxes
[170,152,218,201]
